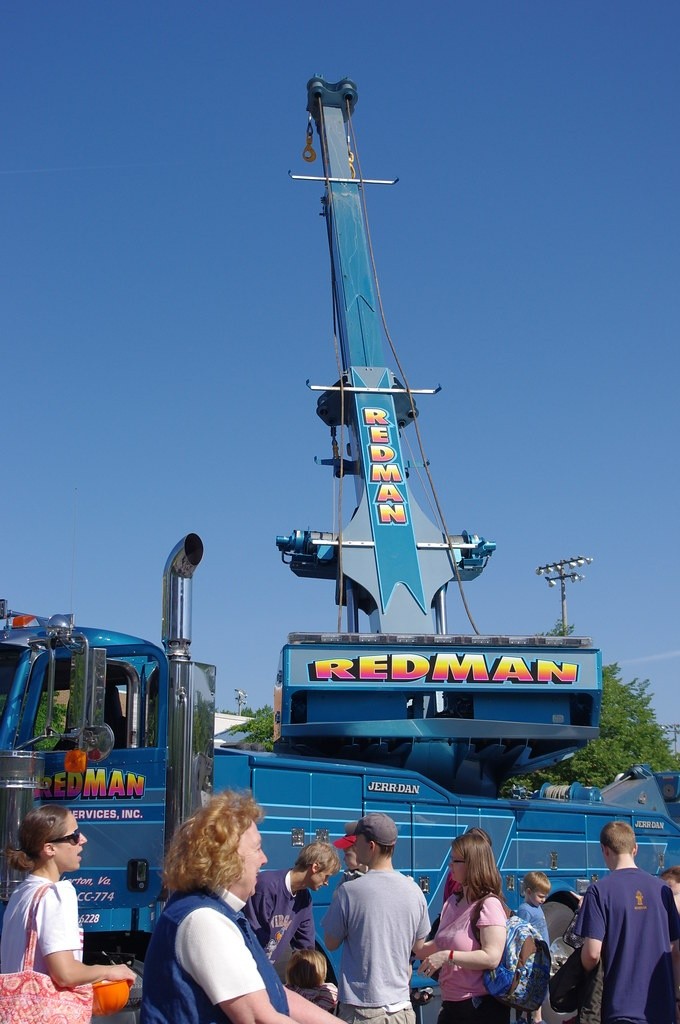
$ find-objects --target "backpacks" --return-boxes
[472,894,551,1011]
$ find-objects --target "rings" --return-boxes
[426,969,430,973]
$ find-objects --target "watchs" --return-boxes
[448,950,455,966]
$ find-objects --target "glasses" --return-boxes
[449,858,465,863]
[46,828,79,843]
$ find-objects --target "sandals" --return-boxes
[410,989,431,1005]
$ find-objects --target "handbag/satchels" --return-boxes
[0,884,93,1024]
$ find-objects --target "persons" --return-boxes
[443,828,492,903]
[334,833,416,981]
[322,813,432,1024]
[517,871,551,1024]
[286,949,338,1011]
[0,804,135,990]
[415,834,509,1024]
[241,842,341,965]
[573,822,680,1024]
[139,790,348,1024]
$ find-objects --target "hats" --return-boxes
[333,835,357,849]
[345,812,397,846]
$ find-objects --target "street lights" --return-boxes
[659,723,680,758]
[532,554,596,640]
[234,688,248,718]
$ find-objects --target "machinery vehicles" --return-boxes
[0,74,680,1024]
